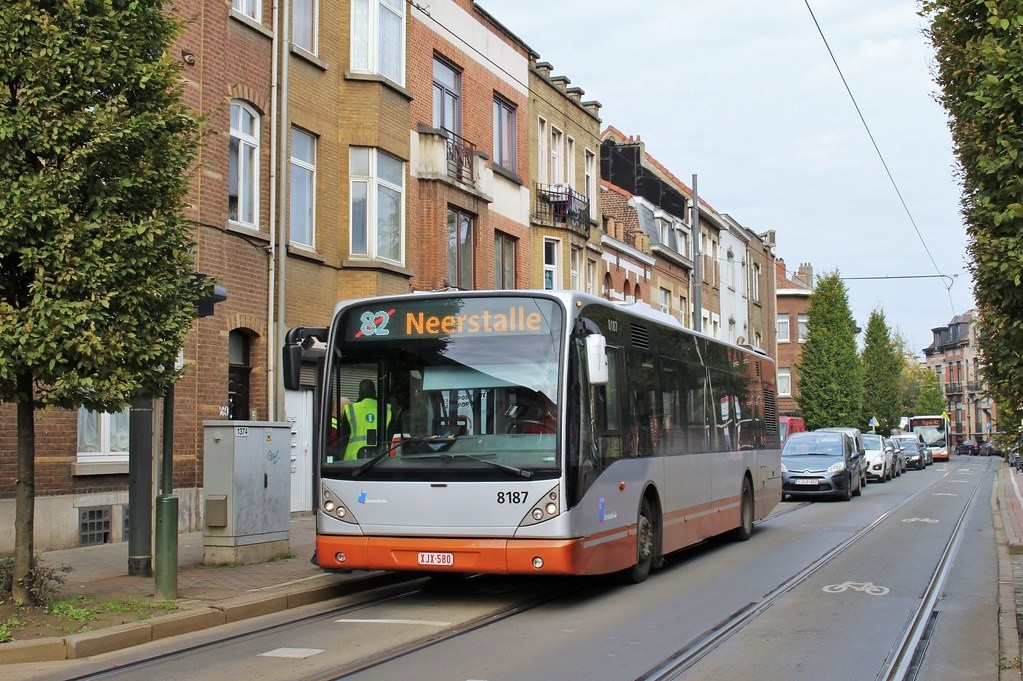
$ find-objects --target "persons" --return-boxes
[521,390,557,434]
[310,396,350,566]
[341,379,397,461]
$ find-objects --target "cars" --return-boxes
[955,440,985,456]
[884,439,909,478]
[923,449,933,465]
[813,427,867,487]
[861,434,892,483]
[890,431,926,470]
[1002,440,1023,471]
[781,431,862,501]
[979,440,1002,456]
[778,416,805,450]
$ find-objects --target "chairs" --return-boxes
[433,416,467,435]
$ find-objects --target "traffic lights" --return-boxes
[214,285,227,303]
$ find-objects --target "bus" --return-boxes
[283,287,782,583]
[908,416,951,462]
